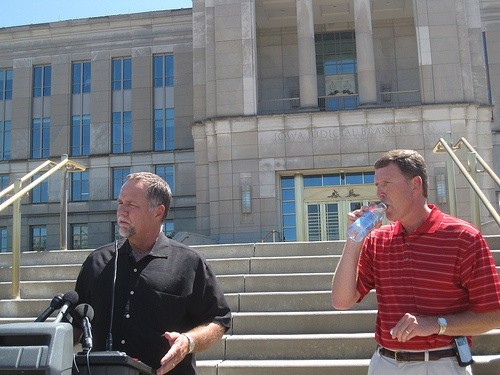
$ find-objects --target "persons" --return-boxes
[57,172,232,375]
[332,149,500,375]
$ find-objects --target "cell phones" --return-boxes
[453,337,472,362]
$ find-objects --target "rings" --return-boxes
[172,363,176,367]
[405,330,410,334]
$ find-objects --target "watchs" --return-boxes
[182,332,195,354]
[436,315,447,335]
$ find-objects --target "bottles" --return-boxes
[346,202,388,242]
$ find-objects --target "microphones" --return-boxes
[34,289,95,349]
[106,239,119,351]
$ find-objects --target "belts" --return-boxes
[380,346,457,361]
